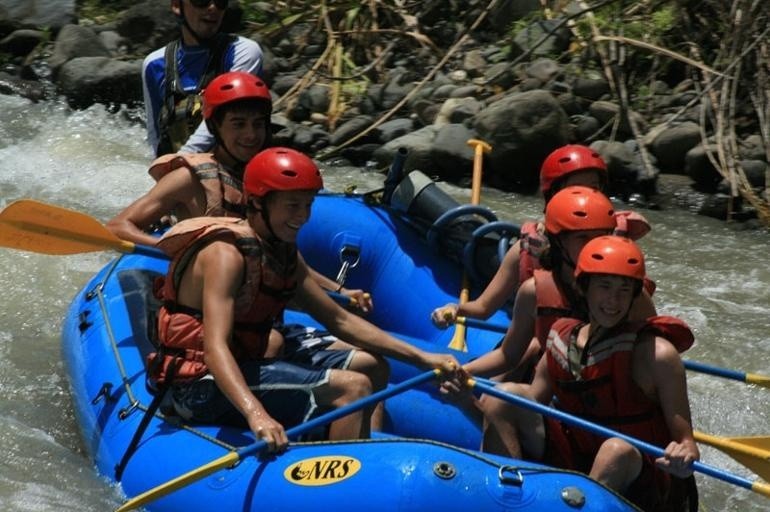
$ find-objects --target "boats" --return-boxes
[62,192,650,511]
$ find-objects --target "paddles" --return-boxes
[447,138,493,351]
[443,314,770,393]
[114,364,445,512]
[0,198,372,310]
[465,373,770,485]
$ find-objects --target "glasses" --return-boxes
[189,0,228,10]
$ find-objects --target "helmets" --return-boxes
[202,71,274,134]
[538,142,607,202]
[542,185,618,239]
[572,231,645,302]
[242,144,324,214]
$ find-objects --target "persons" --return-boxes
[440,234,700,509]
[430,144,652,330]
[140,0,263,151]
[461,185,658,375]
[103,73,375,313]
[148,148,468,454]
